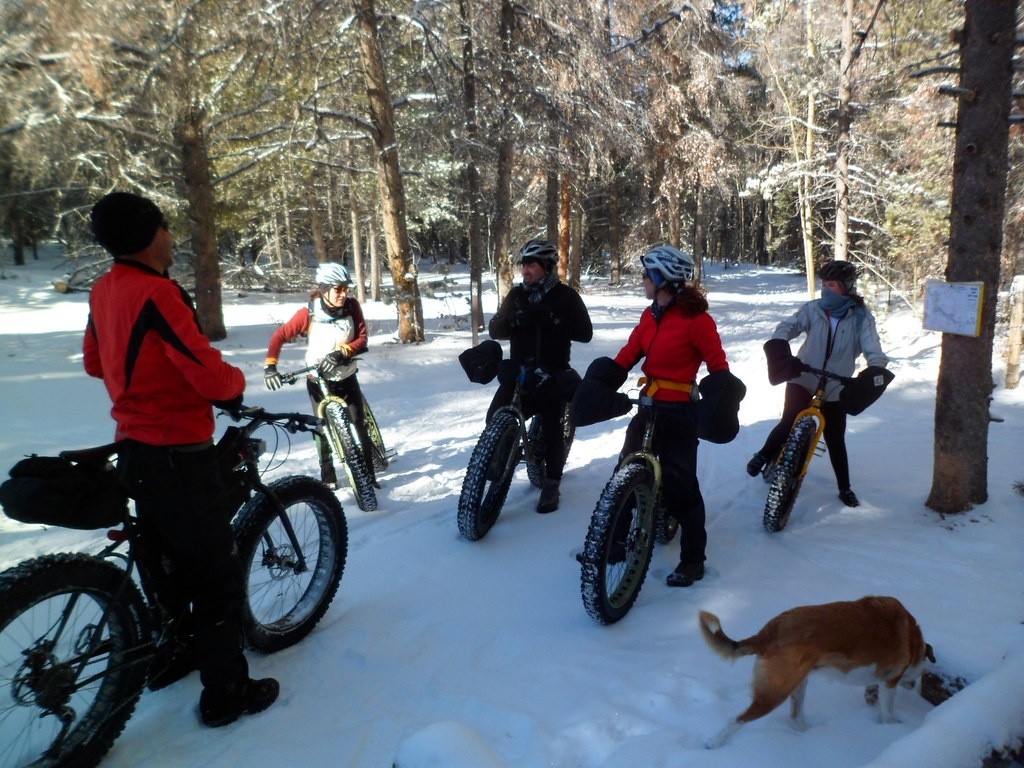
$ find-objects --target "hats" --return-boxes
[91,192,163,257]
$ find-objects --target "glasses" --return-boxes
[331,287,350,293]
[640,267,647,280]
[159,219,169,232]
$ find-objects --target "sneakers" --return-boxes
[322,481,338,491]
[746,449,766,477]
[667,566,704,586]
[202,677,279,727]
[537,485,559,513]
[839,489,860,507]
[372,481,384,490]
[576,549,626,563]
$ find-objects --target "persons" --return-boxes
[486,238,593,514]
[263,261,382,491]
[746,259,891,508]
[568,245,747,588]
[81,190,280,729]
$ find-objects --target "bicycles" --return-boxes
[579,398,690,625]
[0,407,348,768]
[749,365,855,532]
[456,353,578,540]
[278,359,398,511]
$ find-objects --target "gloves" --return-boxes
[320,348,343,374]
[264,364,283,392]
[509,309,529,329]
[543,308,561,329]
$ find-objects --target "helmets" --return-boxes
[316,263,353,285]
[516,240,560,265]
[640,246,694,282]
[818,262,856,281]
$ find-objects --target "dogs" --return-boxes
[699,595,937,749]
